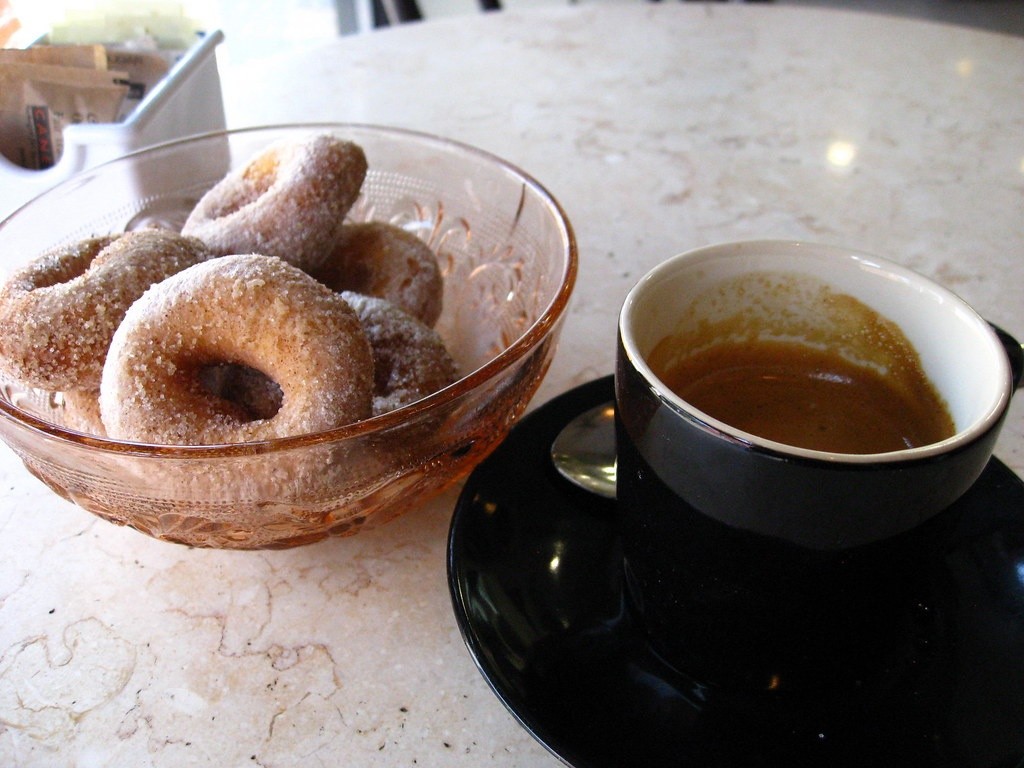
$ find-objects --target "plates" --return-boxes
[445,372,1024,767]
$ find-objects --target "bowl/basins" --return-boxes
[0,116,578,549]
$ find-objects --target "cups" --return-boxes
[615,239,1024,552]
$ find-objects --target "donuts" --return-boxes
[0,133,459,518]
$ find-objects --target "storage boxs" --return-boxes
[0,24,231,264]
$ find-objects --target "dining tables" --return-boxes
[0,2,1024,766]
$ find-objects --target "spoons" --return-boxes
[550,402,616,498]
